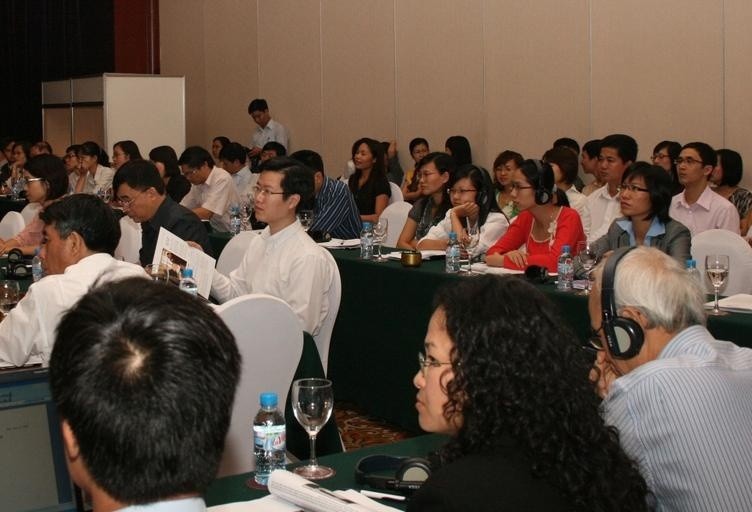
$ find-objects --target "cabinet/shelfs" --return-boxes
[41,72,187,164]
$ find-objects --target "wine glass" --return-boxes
[460,228,481,276]
[299,210,314,231]
[705,253,728,316]
[239,202,252,230]
[372,218,389,263]
[577,240,599,295]
[359,221,373,260]
[291,378,337,480]
[0,279,21,315]
[99,189,111,203]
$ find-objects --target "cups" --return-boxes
[401,252,422,266]
[144,264,169,285]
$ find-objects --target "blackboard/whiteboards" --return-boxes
[104,73,187,162]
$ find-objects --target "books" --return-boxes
[211,468,408,512]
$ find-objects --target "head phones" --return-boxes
[601,246,644,359]
[355,454,433,493]
[8,248,32,276]
[470,164,489,205]
[306,231,331,242]
[527,159,549,205]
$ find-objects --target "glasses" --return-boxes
[649,153,669,161]
[417,350,465,377]
[418,170,440,178]
[21,176,43,184]
[585,325,604,350]
[618,181,649,194]
[250,184,286,196]
[510,181,533,190]
[674,156,705,165]
[446,187,477,195]
[184,166,202,177]
[115,187,149,210]
[64,154,76,159]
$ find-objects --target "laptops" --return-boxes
[0,367,95,512]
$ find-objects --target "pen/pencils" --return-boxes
[356,489,408,503]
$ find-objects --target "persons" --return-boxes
[1,134,752,383]
[47,276,242,511]
[246,99,288,174]
[583,245,751,512]
[407,272,647,512]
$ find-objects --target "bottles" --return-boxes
[557,246,573,291]
[684,258,700,285]
[253,392,287,485]
[178,269,199,297]
[30,248,43,282]
[445,231,460,274]
[12,174,27,201]
[229,207,241,237]
